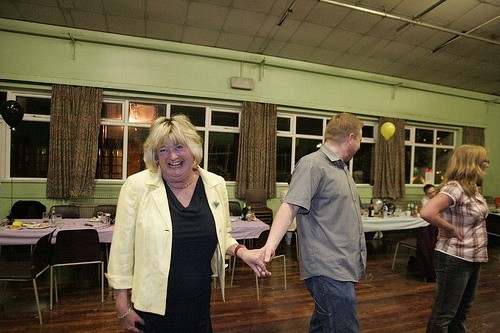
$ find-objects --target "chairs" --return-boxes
[0,199,435,325]
[246,188,274,247]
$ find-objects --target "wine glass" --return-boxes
[97,212,103,224]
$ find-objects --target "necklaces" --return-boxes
[165,169,195,189]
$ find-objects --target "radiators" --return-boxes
[78,206,96,219]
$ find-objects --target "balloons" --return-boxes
[380,122,396,140]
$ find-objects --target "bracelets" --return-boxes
[116,307,131,319]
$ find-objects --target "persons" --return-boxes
[420,144,490,333]
[105,114,267,333]
[421,184,437,207]
[256,112,367,333]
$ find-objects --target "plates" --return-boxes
[2,222,51,231]
[89,218,103,222]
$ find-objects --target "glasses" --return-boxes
[482,161,489,165]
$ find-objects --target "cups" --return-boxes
[6,215,14,225]
[56,214,62,225]
[43,212,49,223]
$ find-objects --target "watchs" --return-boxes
[235,244,246,257]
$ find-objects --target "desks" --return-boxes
[487,206,500,249]
[288,211,430,261]
[0,216,271,298]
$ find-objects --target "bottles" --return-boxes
[50,207,56,228]
[110,212,115,224]
[368,198,375,217]
[406,202,418,217]
[241,202,252,222]
[381,199,388,218]
